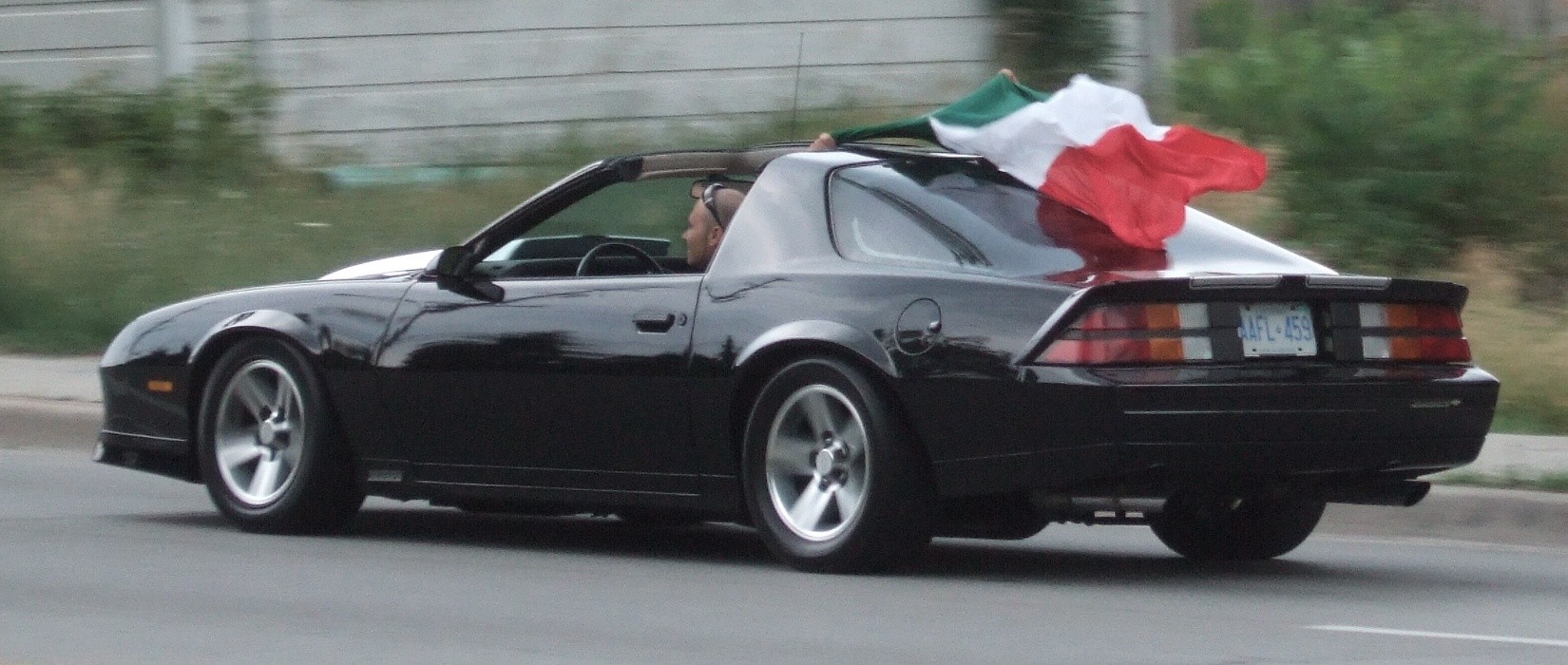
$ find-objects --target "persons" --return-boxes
[680,180,746,271]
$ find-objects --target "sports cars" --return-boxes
[91,32,1497,572]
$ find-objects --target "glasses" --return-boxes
[701,183,726,231]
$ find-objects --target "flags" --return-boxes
[839,71,1269,251]
